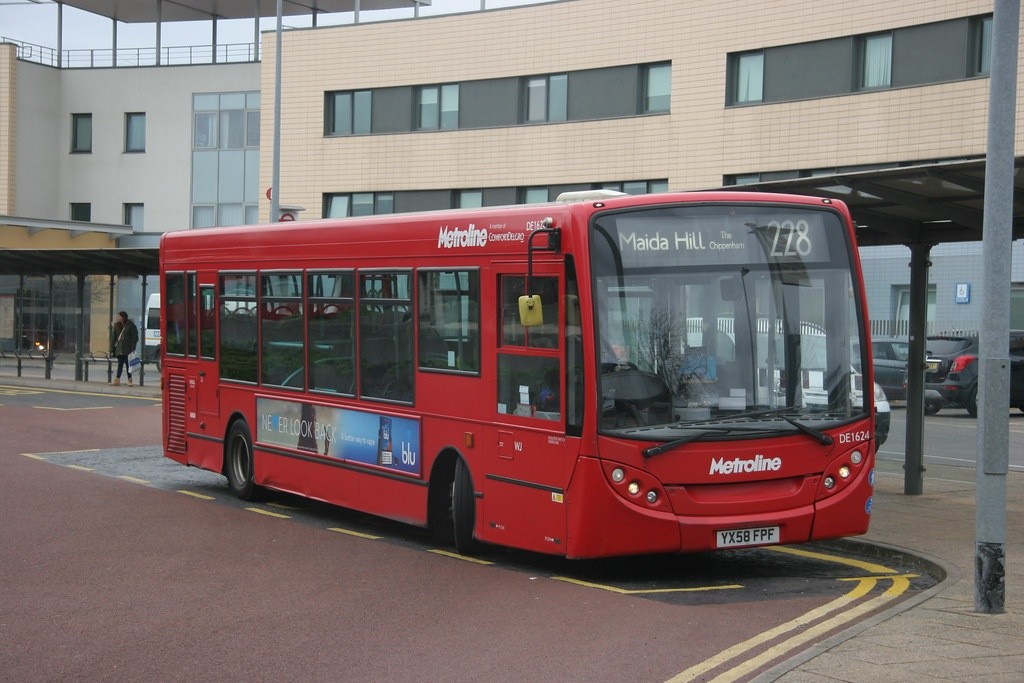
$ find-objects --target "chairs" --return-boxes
[505,363,584,411]
[204,304,474,401]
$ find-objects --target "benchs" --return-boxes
[73,350,118,384]
[0,349,49,378]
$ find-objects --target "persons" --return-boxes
[108,310,140,386]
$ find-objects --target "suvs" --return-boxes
[906,326,1024,418]
[849,335,927,400]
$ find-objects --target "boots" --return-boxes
[107,378,120,386]
[127,378,133,387]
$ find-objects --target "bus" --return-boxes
[142,291,258,373]
[158,189,877,562]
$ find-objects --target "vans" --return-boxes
[683,317,891,455]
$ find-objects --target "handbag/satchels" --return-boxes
[127,351,143,374]
